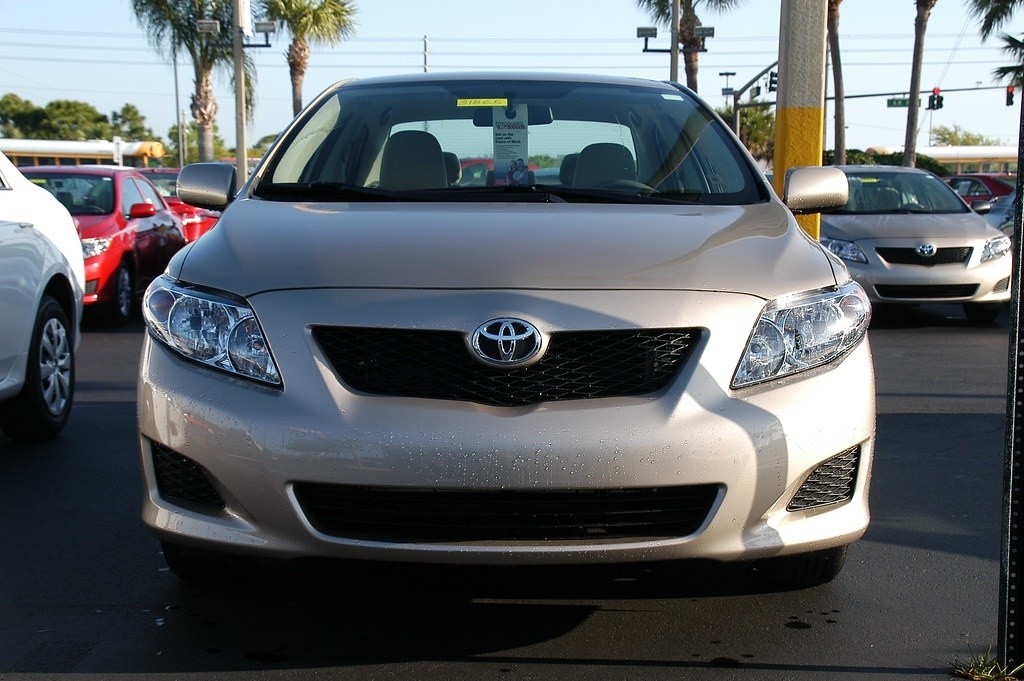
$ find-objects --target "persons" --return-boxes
[506,159,528,186]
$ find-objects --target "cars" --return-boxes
[138,166,221,245]
[982,187,1016,250]
[940,174,1016,206]
[134,73,880,597]
[816,162,1014,329]
[1,147,88,440]
[460,156,540,171]
[16,163,186,329]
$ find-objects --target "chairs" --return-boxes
[574,143,638,188]
[554,153,579,187]
[443,151,463,188]
[97,191,114,212]
[877,190,899,210]
[376,130,448,191]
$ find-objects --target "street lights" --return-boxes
[635,0,715,82]
[194,0,280,192]
[719,71,738,109]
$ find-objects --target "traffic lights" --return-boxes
[1006,85,1014,107]
[928,94,936,109]
[933,87,940,94]
[935,95,943,109]
[765,70,779,93]
